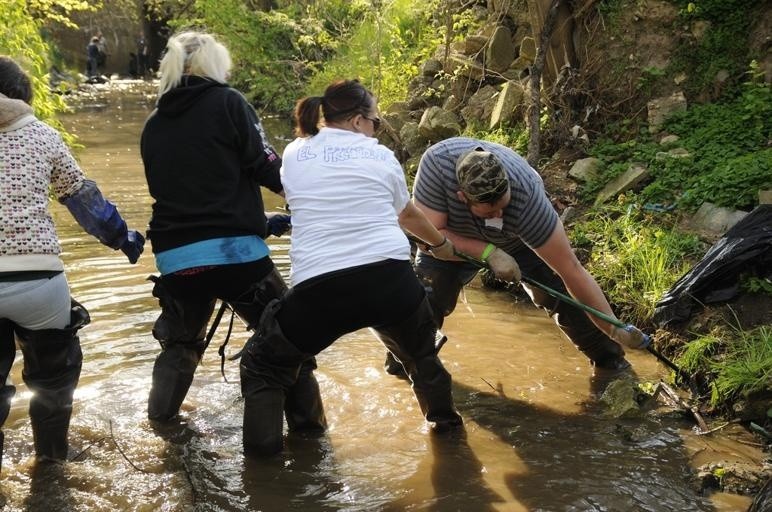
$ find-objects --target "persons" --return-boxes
[0,57,148,465]
[237,77,462,459]
[137,33,329,436]
[384,138,651,387]
[86,36,101,76]
[136,28,154,79]
[97,31,112,75]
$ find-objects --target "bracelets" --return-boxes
[431,236,447,248]
[480,243,494,260]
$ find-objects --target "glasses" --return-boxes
[347,114,381,132]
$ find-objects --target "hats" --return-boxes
[455,148,508,202]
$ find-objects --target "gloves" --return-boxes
[58,180,144,264]
[265,212,292,240]
[486,249,522,282]
[428,237,468,262]
[611,321,649,349]
